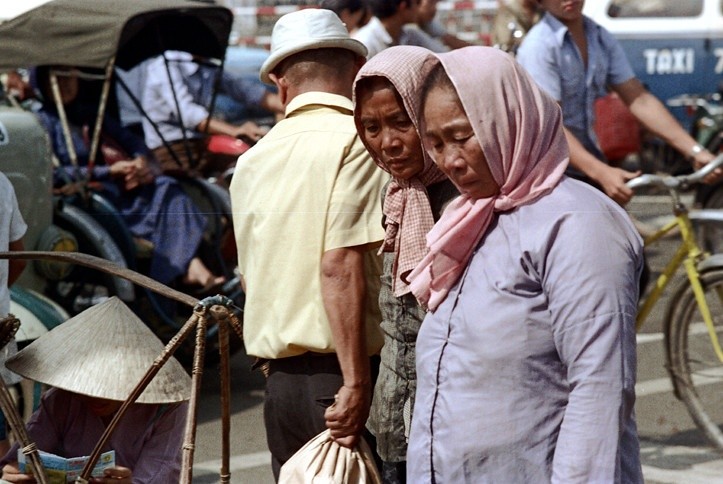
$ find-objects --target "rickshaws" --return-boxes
[0,0,723,457]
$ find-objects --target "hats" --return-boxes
[257,8,368,87]
[4,294,192,405]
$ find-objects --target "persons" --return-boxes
[230,9,392,484]
[0,170,30,462]
[352,46,458,484]
[0,47,283,289]
[407,46,644,484]
[319,0,472,59]
[516,1,723,293]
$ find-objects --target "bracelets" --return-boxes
[687,144,705,160]
